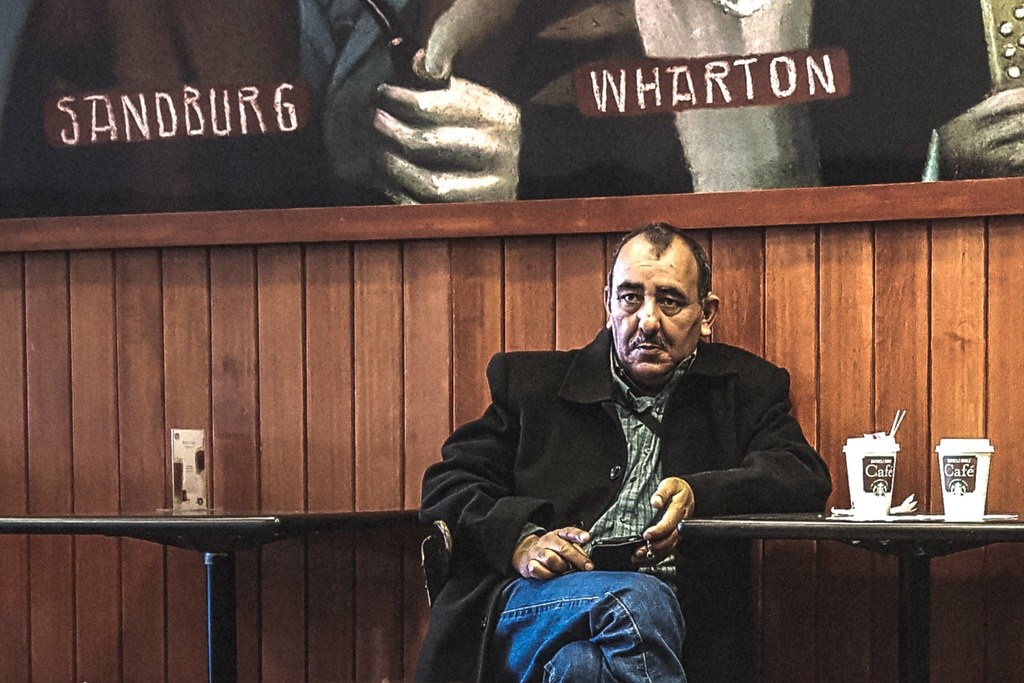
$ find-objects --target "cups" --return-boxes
[844,436,901,518]
[935,437,996,524]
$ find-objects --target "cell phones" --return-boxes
[590,535,641,547]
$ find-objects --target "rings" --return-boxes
[677,521,685,535]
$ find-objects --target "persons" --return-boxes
[411,222,832,683]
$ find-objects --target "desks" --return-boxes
[0,509,421,683]
[678,512,1024,682]
